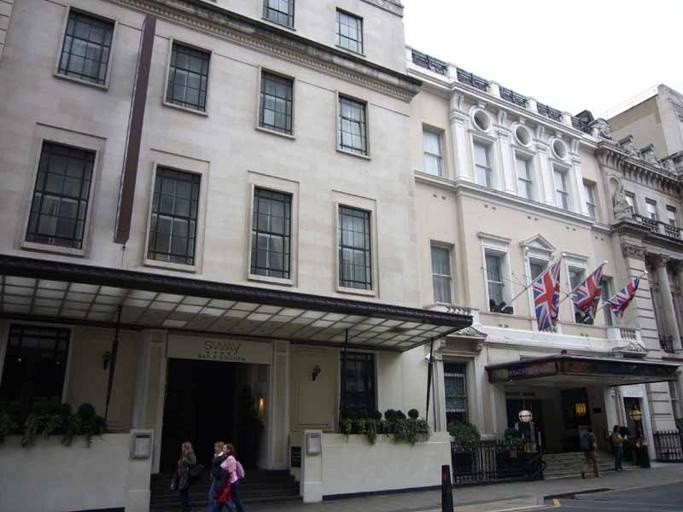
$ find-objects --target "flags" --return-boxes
[571,265,603,321]
[531,258,560,331]
[604,275,640,321]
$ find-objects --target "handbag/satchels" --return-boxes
[189,463,205,478]
[236,461,245,481]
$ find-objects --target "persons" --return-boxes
[169,441,196,511]
[212,444,246,512]
[580,427,603,479]
[628,403,643,438]
[609,425,627,472]
[206,442,236,511]
